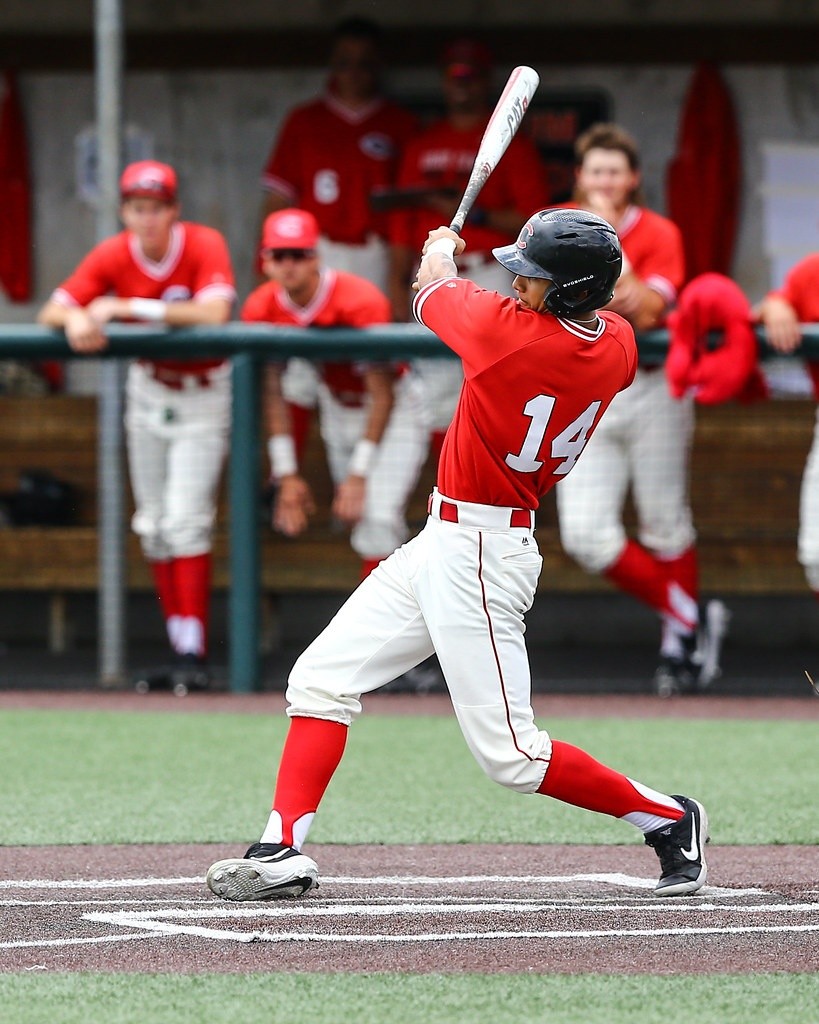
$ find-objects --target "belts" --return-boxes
[428,494,530,527]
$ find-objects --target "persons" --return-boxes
[206,208,708,901]
[240,209,442,686]
[256,24,546,580]
[38,161,237,692]
[546,124,729,699]
[761,253,819,601]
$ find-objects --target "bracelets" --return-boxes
[348,441,376,477]
[269,437,296,476]
[132,297,165,321]
[425,238,456,260]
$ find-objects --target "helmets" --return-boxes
[491,208,622,317]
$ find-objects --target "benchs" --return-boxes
[0,396,819,656]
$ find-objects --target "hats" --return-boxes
[438,38,491,77]
[261,209,319,251]
[118,159,179,200]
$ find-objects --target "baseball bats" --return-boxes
[449,65,540,236]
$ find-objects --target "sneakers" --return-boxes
[206,842,321,902]
[643,794,708,896]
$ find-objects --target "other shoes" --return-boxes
[655,598,726,696]
[141,646,213,693]
